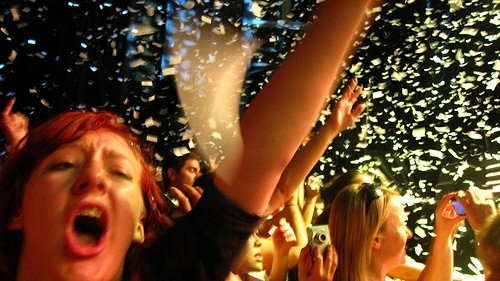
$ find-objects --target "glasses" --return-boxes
[365,182,382,216]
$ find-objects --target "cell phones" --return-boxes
[450,196,466,216]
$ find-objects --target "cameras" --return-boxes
[306,224,331,256]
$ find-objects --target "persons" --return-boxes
[227,78,500,281]
[0,97,222,245]
[0,0,372,281]
